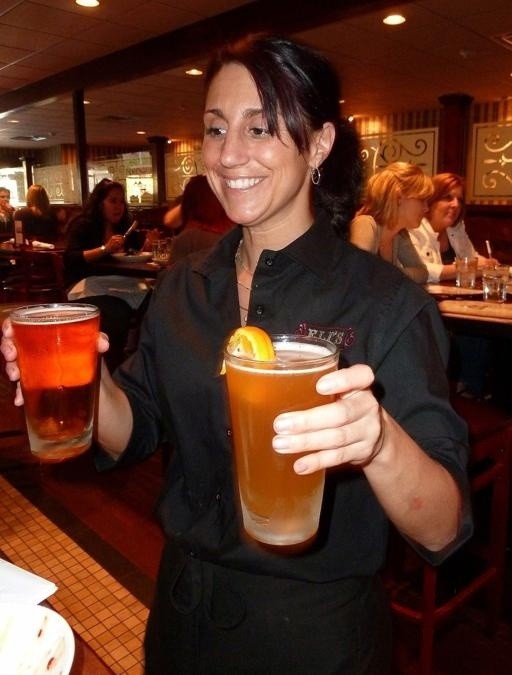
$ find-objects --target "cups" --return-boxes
[152,240,169,262]
[222,333,339,547]
[455,255,509,304]
[10,304,101,462]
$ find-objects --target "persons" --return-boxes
[396,171,500,284]
[350,161,435,284]
[0,33,480,675]
[0,176,232,365]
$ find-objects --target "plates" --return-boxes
[110,252,153,263]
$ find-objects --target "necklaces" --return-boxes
[234,239,257,311]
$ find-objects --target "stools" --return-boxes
[382,400,511,675]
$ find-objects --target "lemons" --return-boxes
[226,326,274,362]
